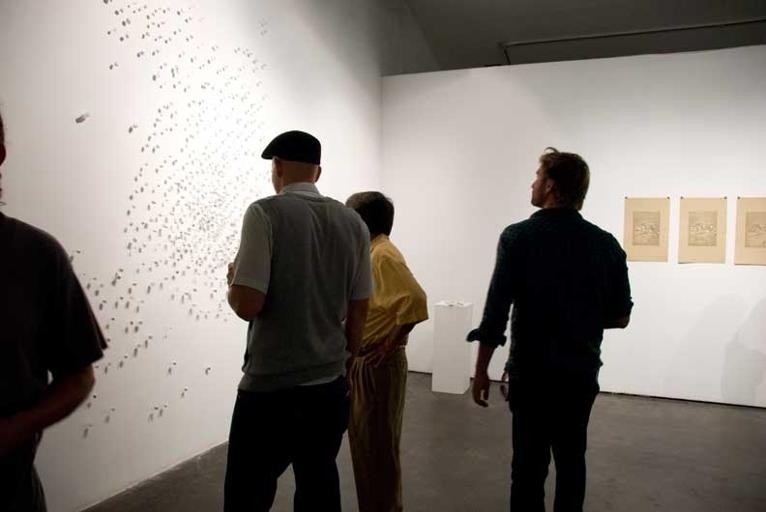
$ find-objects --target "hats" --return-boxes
[261,131,321,165]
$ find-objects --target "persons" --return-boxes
[344,191,428,512]
[0,116,108,512]
[467,145,634,512]
[223,131,372,512]
